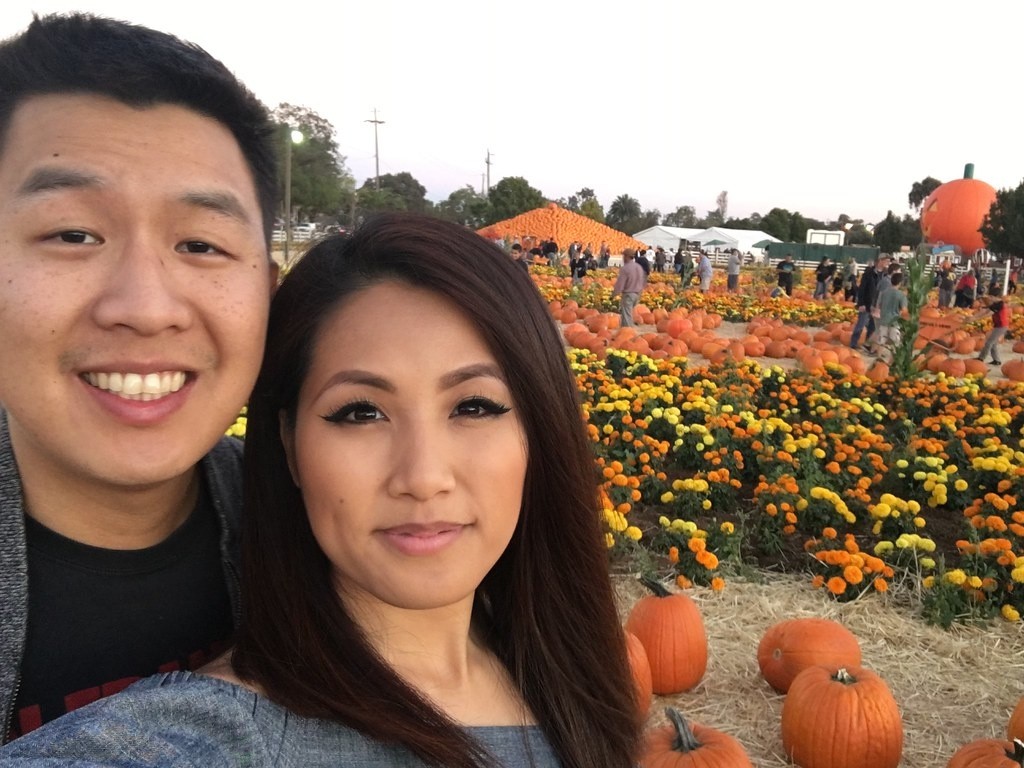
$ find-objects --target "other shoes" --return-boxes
[988,360,1001,365]
[973,358,983,362]
[867,350,879,357]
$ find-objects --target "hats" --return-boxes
[878,253,894,259]
[621,248,635,257]
[951,264,958,268]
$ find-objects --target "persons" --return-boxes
[813,254,838,300]
[0,13,283,745]
[635,246,685,274]
[773,254,796,297]
[509,237,558,275]
[694,249,712,294]
[569,240,612,286]
[0,213,637,767]
[726,249,743,292]
[832,253,1020,365]
[612,249,645,327]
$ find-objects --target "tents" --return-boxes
[687,226,784,266]
[632,224,706,250]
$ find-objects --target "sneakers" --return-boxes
[861,344,873,353]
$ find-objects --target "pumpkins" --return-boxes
[614,573,1024,768]
[527,273,1024,382]
[478,201,648,262]
